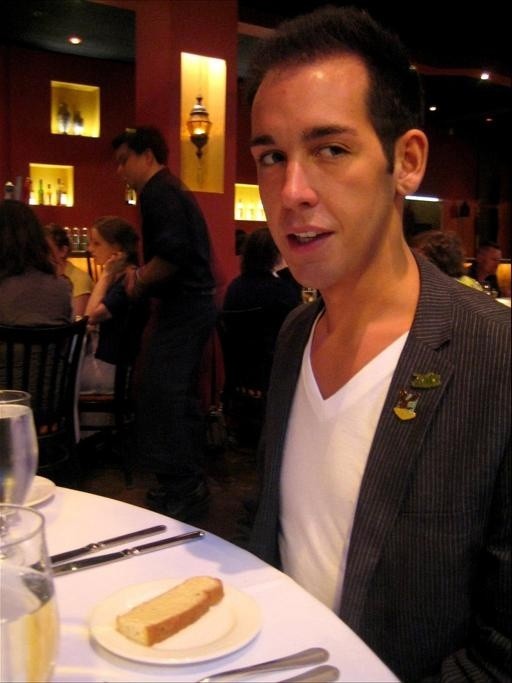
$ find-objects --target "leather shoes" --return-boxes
[162,483,208,521]
[145,487,170,507]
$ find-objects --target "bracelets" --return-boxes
[135,269,149,285]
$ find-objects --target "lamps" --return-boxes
[186,96,213,158]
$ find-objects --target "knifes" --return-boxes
[29,523,204,576]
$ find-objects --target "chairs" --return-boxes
[78,295,147,487]
[215,307,273,469]
[0,314,90,486]
[84,251,104,283]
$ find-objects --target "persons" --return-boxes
[0,201,74,429]
[78,215,145,396]
[465,240,503,297]
[411,229,484,292]
[229,3,512,683]
[113,126,219,524]
[42,223,97,318]
[215,228,302,311]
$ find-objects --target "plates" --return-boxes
[23,475,55,508]
[87,580,261,666]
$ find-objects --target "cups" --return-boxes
[1,502,63,683]
[1,388,39,504]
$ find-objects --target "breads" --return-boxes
[114,575,225,648]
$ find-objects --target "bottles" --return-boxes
[2,175,64,206]
[57,98,83,136]
[65,225,89,251]
